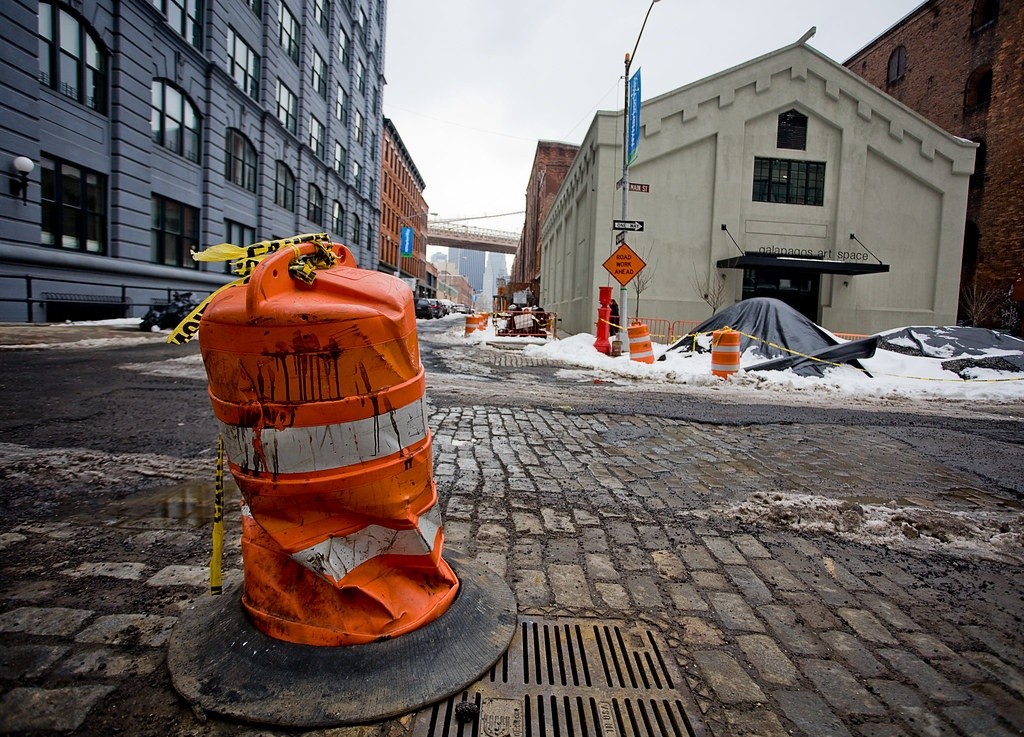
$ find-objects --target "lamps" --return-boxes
[9,157,35,207]
[381,232,391,241]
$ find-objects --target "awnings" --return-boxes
[717,255,890,277]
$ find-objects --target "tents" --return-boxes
[657,297,1024,378]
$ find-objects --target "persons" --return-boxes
[609,299,619,337]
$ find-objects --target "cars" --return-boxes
[414,298,471,320]
